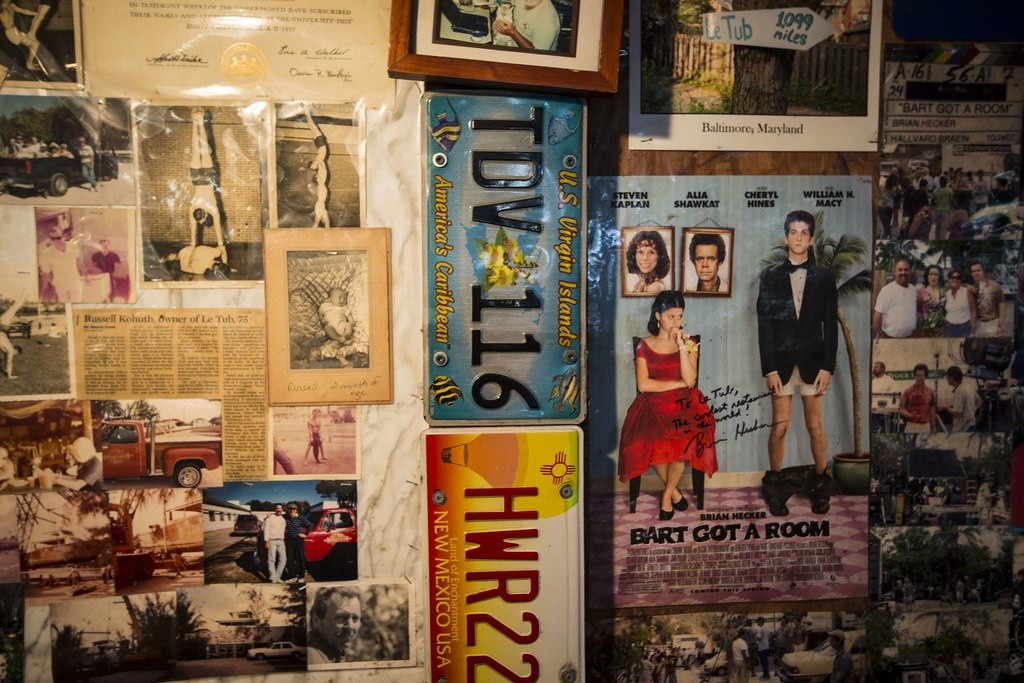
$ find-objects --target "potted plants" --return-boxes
[759,209,870,495]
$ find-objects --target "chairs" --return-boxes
[630,335,704,514]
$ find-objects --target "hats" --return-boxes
[17,136,22,138]
[79,138,85,141]
[288,504,297,508]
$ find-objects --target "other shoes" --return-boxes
[672,487,688,510]
[659,509,674,520]
[286,578,298,583]
[272,580,276,583]
[90,187,94,192]
[813,503,829,514]
[770,500,788,516]
[760,675,770,679]
[270,576,283,583]
[95,185,100,191]
[299,579,304,582]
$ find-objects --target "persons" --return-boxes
[620,149,1024,683]
[0,408,363,683]
[491,0,562,53]
[0,0,369,384]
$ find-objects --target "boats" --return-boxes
[25,527,105,568]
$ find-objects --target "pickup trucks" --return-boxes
[255,508,356,570]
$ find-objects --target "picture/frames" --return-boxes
[388,0,623,93]
[622,226,675,298]
[262,228,394,406]
[680,227,734,298]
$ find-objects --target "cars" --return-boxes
[776,629,867,683]
[233,515,261,534]
[247,642,307,661]
[704,651,728,677]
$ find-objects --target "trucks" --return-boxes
[0,143,118,197]
[93,419,222,488]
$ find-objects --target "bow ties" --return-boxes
[789,262,807,273]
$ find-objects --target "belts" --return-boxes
[908,420,927,424]
[977,317,997,322]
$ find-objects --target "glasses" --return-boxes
[51,236,62,240]
[951,276,960,279]
